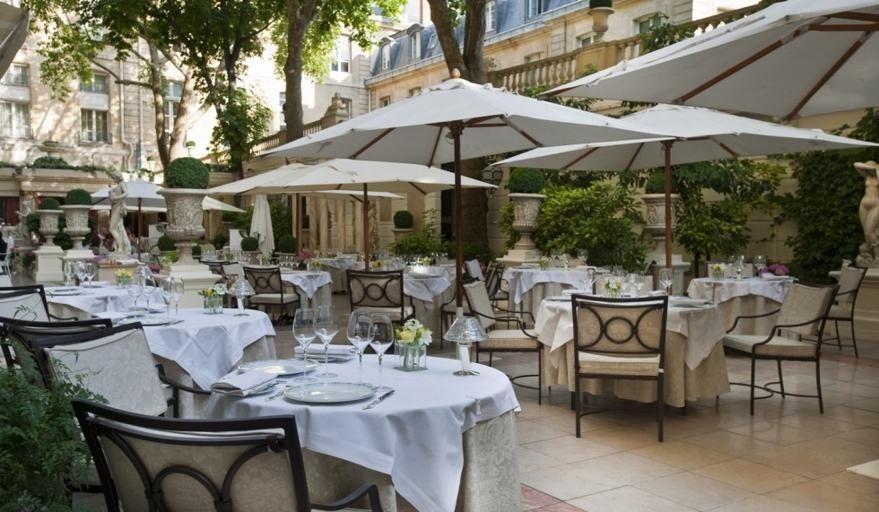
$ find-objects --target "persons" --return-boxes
[109,172,131,263]
[853,161,878,267]
[0,230,15,274]
[14,201,32,239]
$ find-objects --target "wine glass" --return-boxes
[64,258,97,290]
[593,266,675,298]
[126,275,186,323]
[548,246,589,270]
[727,254,766,282]
[345,309,374,385]
[312,302,341,384]
[204,239,448,274]
[369,310,395,388]
[290,307,318,382]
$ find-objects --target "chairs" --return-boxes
[486,256,513,326]
[648,262,688,295]
[4,313,109,398]
[457,276,555,405]
[243,266,301,335]
[716,280,840,414]
[808,266,868,358]
[341,267,419,357]
[571,293,670,446]
[437,259,486,348]
[33,322,178,424]
[3,285,55,331]
[74,399,387,512]
[215,261,243,306]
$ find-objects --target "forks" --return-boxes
[265,385,294,401]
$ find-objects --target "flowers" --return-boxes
[395,320,431,349]
[114,269,130,277]
[198,287,216,298]
[602,281,623,292]
[710,264,724,270]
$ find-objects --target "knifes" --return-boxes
[114,313,141,322]
[172,320,184,325]
[365,390,398,410]
[547,298,571,303]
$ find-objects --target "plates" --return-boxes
[124,315,167,325]
[284,381,374,404]
[237,357,316,377]
[43,288,81,296]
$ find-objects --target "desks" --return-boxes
[498,259,595,331]
[41,272,180,324]
[538,287,730,423]
[688,268,795,342]
[206,352,523,512]
[79,308,281,392]
[232,264,336,321]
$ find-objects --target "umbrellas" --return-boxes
[90,181,168,262]
[203,162,407,256]
[250,68,683,321]
[535,0,879,124]
[232,157,500,274]
[250,194,276,265]
[487,102,878,295]
[202,196,247,240]
